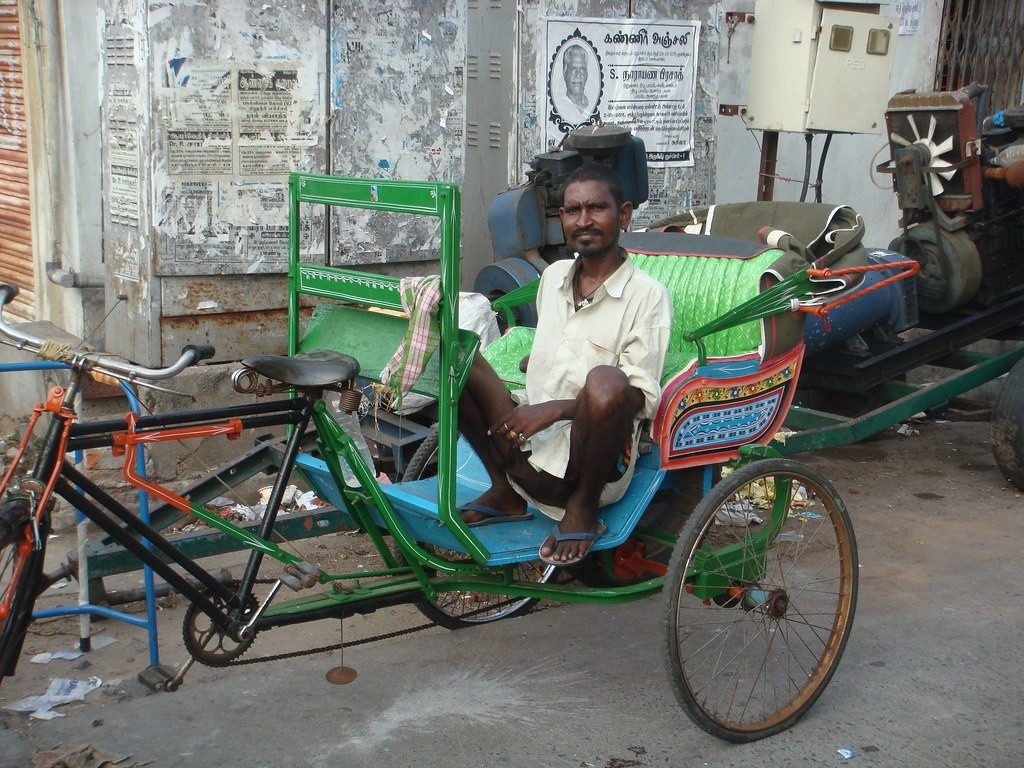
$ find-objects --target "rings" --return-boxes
[509,430,518,440]
[503,423,510,431]
[518,432,528,443]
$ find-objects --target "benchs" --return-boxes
[483,232,788,420]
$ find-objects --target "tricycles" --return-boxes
[2,174,919,744]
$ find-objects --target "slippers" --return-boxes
[456,502,533,528]
[538,522,607,566]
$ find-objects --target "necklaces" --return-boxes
[573,248,643,311]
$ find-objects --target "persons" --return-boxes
[433,162,674,572]
